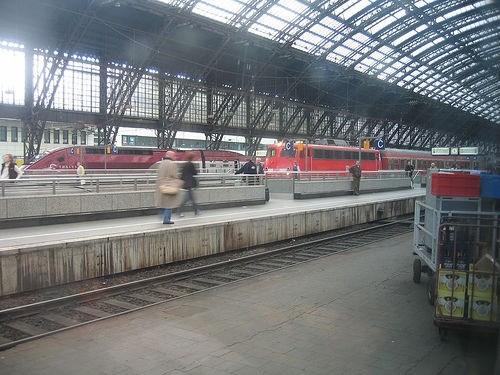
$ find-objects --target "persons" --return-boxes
[430,162,438,183]
[234,156,259,204]
[0,153,22,208]
[348,161,362,196]
[154,151,185,225]
[252,158,265,204]
[176,152,202,218]
[405,160,415,188]
[76,162,85,187]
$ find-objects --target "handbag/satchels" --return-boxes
[159,183,179,194]
[179,178,197,189]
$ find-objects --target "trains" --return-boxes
[14,144,255,180]
[262,142,499,184]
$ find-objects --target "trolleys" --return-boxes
[412,167,500,343]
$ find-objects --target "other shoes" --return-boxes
[180,213,184,217]
[163,219,174,224]
